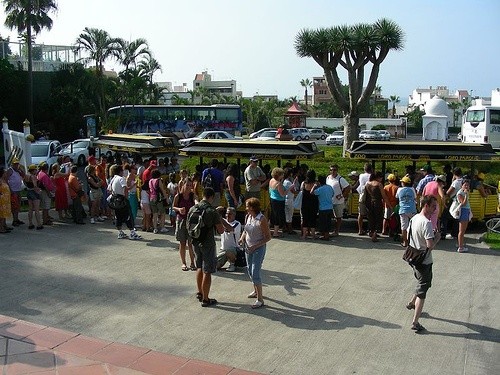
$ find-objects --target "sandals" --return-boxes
[182,264,196,271]
[406,302,415,310]
[411,322,424,330]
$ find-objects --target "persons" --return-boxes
[202,159,224,206]
[0,155,87,233]
[87,136,96,157]
[173,177,199,271]
[216,206,242,271]
[238,197,271,308]
[193,161,272,187]
[83,154,188,234]
[268,162,350,241]
[224,163,241,207]
[243,157,266,200]
[357,163,463,246]
[348,171,360,193]
[406,195,441,332]
[464,169,492,197]
[107,165,144,239]
[193,187,224,307]
[277,125,288,134]
[455,179,471,253]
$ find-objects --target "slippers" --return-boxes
[248,291,264,308]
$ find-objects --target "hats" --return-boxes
[12,158,47,171]
[348,171,359,176]
[250,155,259,161]
[150,160,156,167]
[387,165,432,184]
[88,156,96,162]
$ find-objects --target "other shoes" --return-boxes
[226,263,236,272]
[197,293,216,307]
[272,230,408,247]
[0,215,111,233]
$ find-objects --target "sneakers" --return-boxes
[118,226,169,239]
[458,247,469,252]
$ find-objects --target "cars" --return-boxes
[308,129,327,140]
[293,128,311,140]
[457,132,461,141]
[359,130,367,139]
[249,128,276,139]
[377,130,390,141]
[325,131,344,145]
[59,138,98,167]
[287,129,302,141]
[178,131,243,146]
[364,131,381,141]
[251,131,277,140]
[31,139,62,175]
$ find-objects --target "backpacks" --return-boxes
[187,204,210,238]
[204,169,216,191]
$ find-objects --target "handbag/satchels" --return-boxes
[109,195,127,209]
[449,201,461,219]
[402,245,427,265]
[295,191,303,210]
[234,247,247,267]
[394,204,399,214]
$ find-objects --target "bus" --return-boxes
[107,104,242,139]
[461,106,500,152]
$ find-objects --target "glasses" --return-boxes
[253,160,258,163]
[330,169,336,171]
